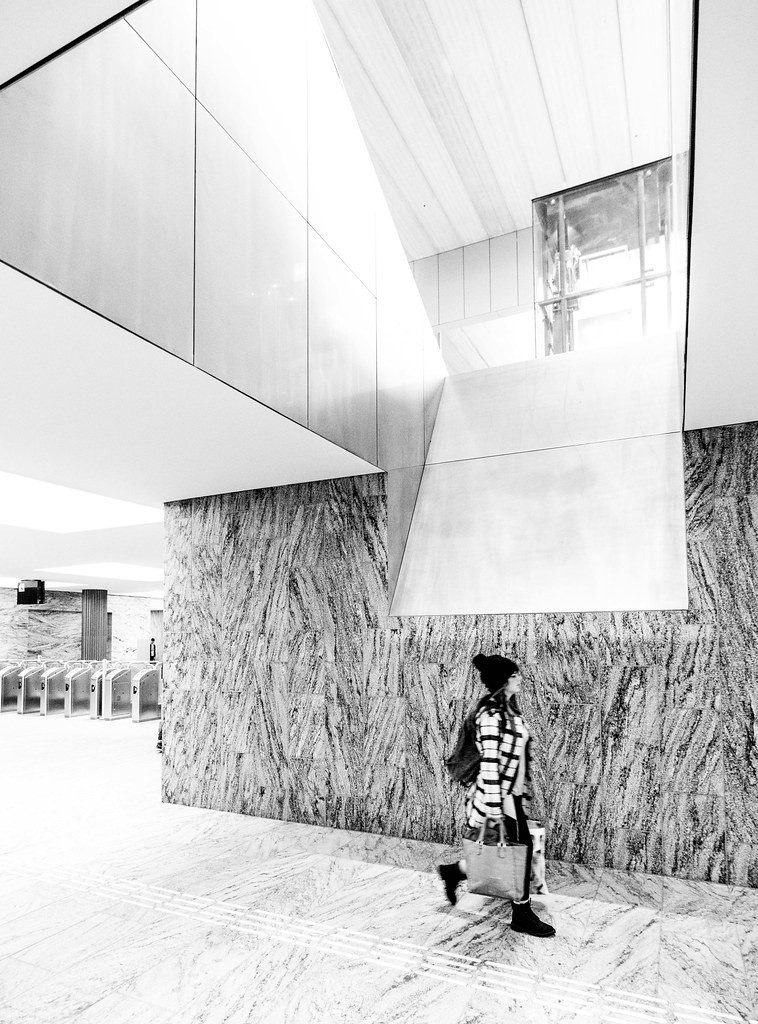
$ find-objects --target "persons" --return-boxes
[156,729,162,751]
[437,654,556,938]
[150,638,156,661]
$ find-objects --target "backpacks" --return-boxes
[446,704,507,787]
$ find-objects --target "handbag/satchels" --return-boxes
[525,820,548,895]
[461,819,528,902]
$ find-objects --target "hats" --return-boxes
[471,654,520,694]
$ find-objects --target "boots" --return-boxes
[438,861,467,906]
[511,901,555,936]
